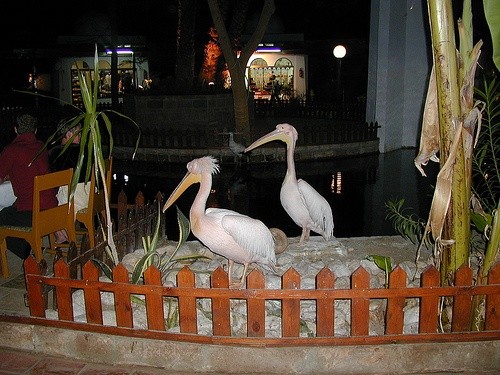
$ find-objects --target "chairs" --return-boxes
[50,157,112,256]
[1,168,77,278]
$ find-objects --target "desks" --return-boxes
[1,181,98,222]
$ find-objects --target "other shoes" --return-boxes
[31,244,46,260]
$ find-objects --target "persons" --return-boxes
[48,117,98,250]
[270,75,281,102]
[0,113,59,262]
[118,73,151,94]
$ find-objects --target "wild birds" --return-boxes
[246,123,334,243]
[218,130,245,157]
[162,155,279,292]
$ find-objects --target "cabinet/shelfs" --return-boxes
[72,68,136,100]
